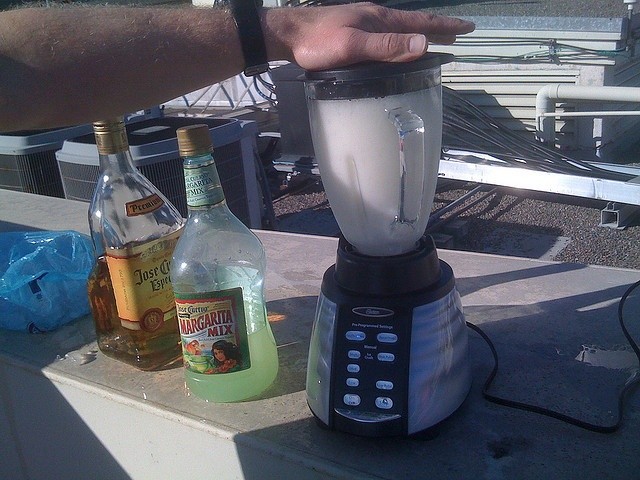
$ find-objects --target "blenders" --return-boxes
[295,53,473,444]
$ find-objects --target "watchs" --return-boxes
[212,0,269,77]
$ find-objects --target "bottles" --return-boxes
[86,114,184,372]
[169,124,279,402]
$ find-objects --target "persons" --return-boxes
[0,0,475,134]
